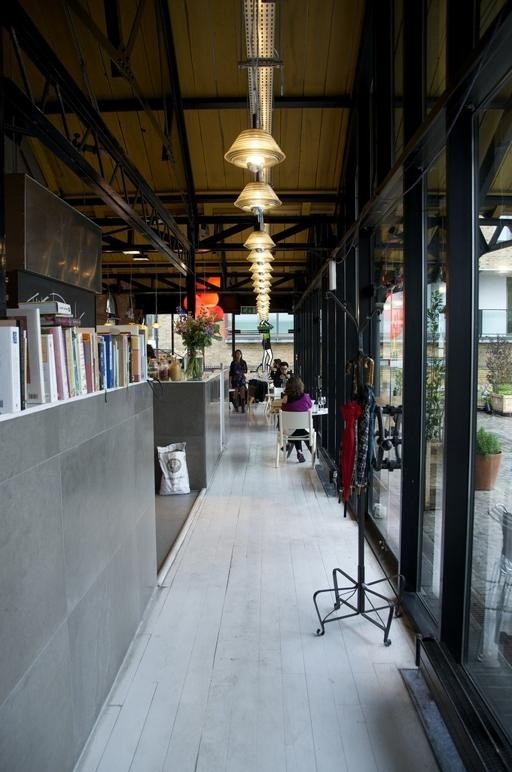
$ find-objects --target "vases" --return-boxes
[185,346,206,380]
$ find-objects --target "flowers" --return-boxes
[173,303,224,381]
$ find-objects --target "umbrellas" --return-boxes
[336,355,376,520]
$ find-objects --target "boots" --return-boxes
[233,398,246,414]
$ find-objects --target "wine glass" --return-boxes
[318,396,326,411]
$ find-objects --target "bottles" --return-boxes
[149,354,182,381]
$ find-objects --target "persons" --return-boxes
[271,358,281,379]
[281,375,319,462]
[229,349,248,413]
[273,362,293,387]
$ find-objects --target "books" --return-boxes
[0,301,147,414]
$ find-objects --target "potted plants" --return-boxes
[475,424,503,492]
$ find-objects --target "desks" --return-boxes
[265,391,328,459]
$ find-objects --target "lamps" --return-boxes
[223,129,288,322]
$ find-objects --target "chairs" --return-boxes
[272,384,319,470]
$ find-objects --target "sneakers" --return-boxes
[281,443,305,462]
